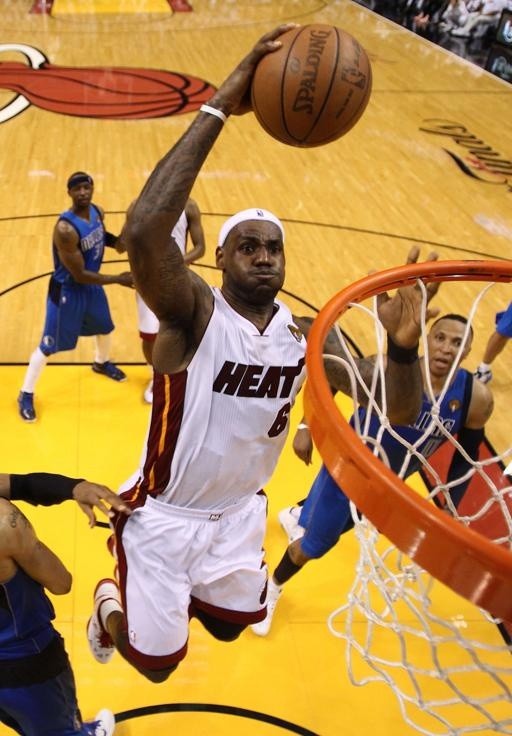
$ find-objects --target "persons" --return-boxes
[356,0,511,44]
[470,299,512,385]
[117,191,205,404]
[84,20,444,683]
[244,312,494,639]
[399,19,510,84]
[16,172,135,420]
[1,470,134,736]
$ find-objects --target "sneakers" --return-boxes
[92,361,126,382]
[474,368,492,384]
[17,392,36,421]
[251,576,282,636]
[144,388,153,403]
[279,506,304,541]
[86,579,120,665]
[87,710,115,736]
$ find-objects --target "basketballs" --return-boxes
[247,24,374,149]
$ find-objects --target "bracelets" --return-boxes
[386,329,421,364]
[195,101,232,124]
[296,424,313,432]
